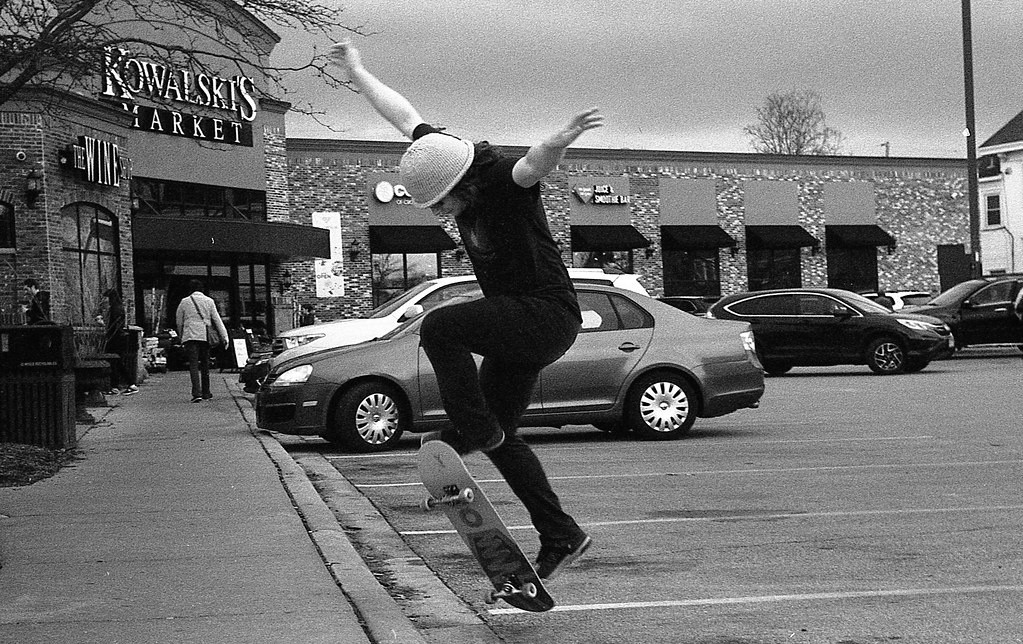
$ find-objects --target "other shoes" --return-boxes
[190,393,214,403]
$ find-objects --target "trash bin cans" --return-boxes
[0,325,77,450]
[119,326,144,384]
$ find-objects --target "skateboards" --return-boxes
[416,440,555,613]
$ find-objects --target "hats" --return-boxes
[400,132,474,209]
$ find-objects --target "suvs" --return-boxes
[828,290,933,318]
[651,294,713,320]
[268,267,656,372]
[704,287,953,375]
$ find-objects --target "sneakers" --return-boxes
[104,385,140,397]
[421,423,594,582]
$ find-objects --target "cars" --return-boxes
[252,283,767,454]
[891,270,1023,358]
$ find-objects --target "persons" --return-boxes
[299,305,316,327]
[99,288,139,397]
[175,278,230,402]
[20,277,51,328]
[874,288,895,314]
[328,40,607,588]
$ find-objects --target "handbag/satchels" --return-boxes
[206,324,221,347]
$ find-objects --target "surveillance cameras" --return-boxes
[16,151,27,161]
